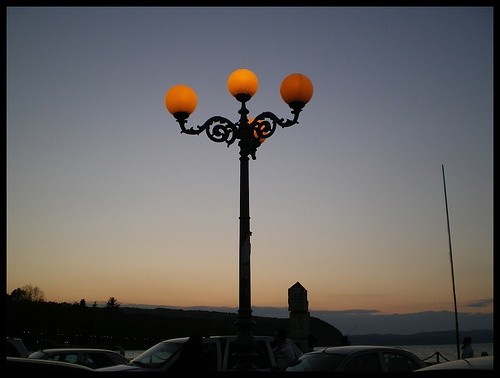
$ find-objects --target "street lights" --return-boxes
[165,68,313,370]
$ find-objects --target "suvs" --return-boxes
[94,333,303,374]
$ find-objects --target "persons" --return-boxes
[271,327,304,374]
[460,336,475,360]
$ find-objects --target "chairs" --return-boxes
[387,357,414,377]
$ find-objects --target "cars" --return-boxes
[27,348,132,371]
[284,344,426,372]
